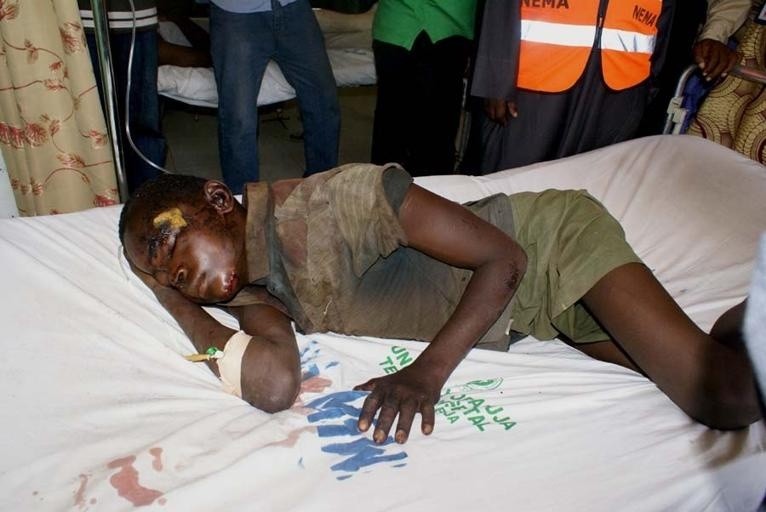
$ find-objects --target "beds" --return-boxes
[1,62,766,512]
[148,1,390,109]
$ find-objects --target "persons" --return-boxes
[207,1,341,196]
[80,1,166,199]
[119,162,765,445]
[470,1,764,172]
[370,1,477,177]
